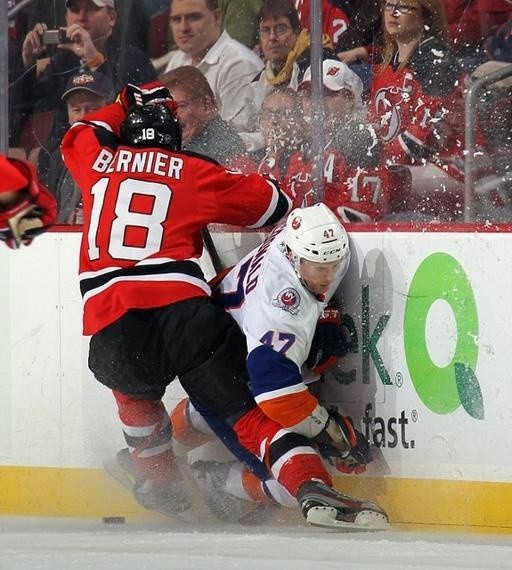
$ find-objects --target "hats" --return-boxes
[60,70,117,101]
[296,59,352,92]
[65,0,116,9]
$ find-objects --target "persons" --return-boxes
[169,201,377,525]
[60,79,389,522]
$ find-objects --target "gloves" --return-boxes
[315,409,373,474]
[0,157,57,249]
[121,81,178,118]
[307,306,343,375]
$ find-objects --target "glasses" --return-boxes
[381,0,419,14]
[256,26,293,38]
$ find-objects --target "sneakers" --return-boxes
[295,479,389,525]
[116,448,190,514]
[208,459,272,528]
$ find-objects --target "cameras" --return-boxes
[39,30,66,44]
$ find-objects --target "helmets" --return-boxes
[282,202,351,282]
[120,102,182,152]
